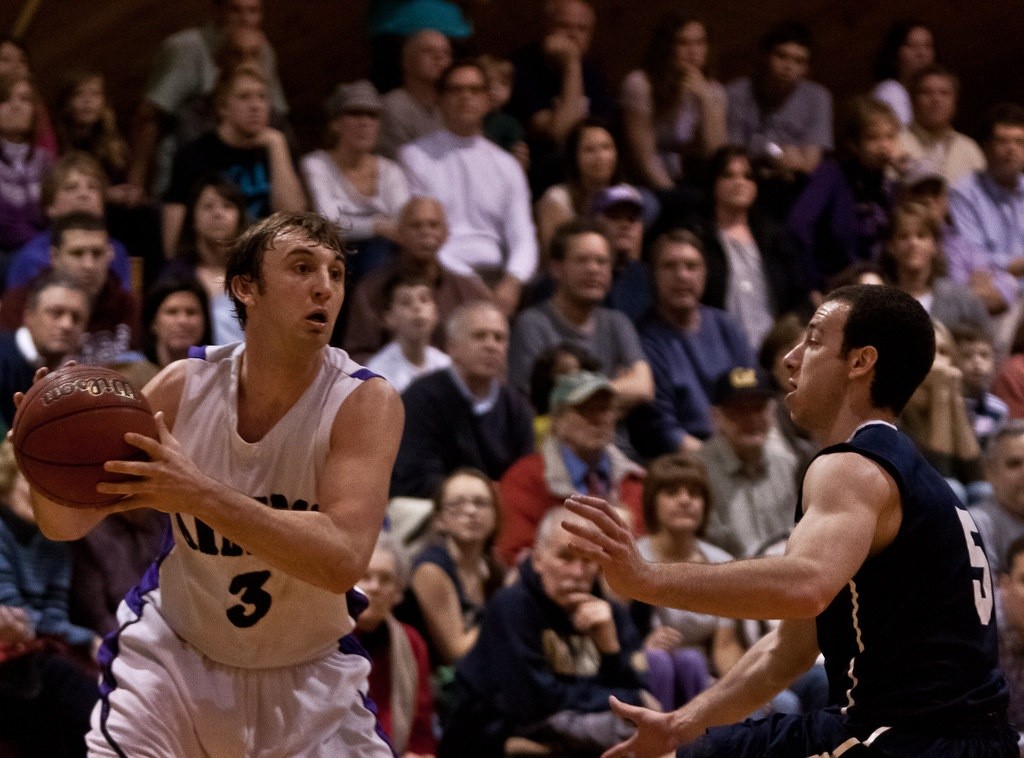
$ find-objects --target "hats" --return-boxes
[549,368,620,410]
[710,365,778,410]
[327,79,385,119]
[594,181,646,214]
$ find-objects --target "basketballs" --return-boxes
[13,364,156,511]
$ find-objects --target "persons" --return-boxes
[0,0,1024,758]
